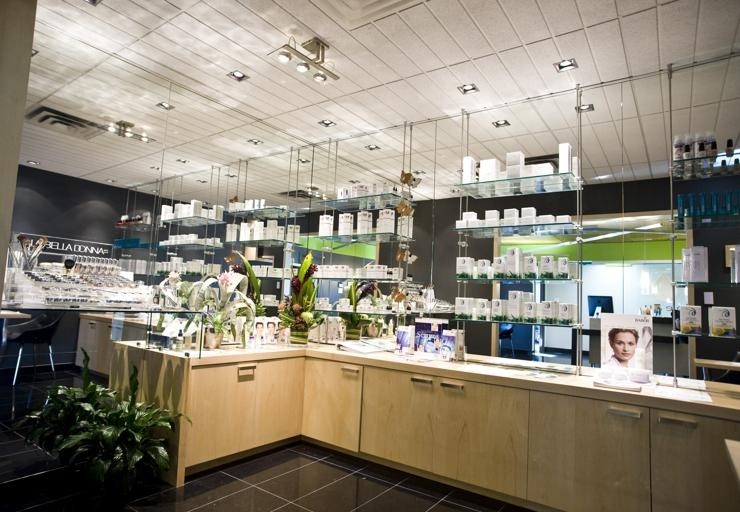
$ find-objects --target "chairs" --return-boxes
[5,309,68,388]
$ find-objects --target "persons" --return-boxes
[602,328,643,369]
[254,322,289,344]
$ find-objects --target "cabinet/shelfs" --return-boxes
[453,147,584,379]
[183,356,305,468]
[527,390,740,510]
[108,211,152,287]
[359,364,528,501]
[304,182,415,352]
[152,201,219,342]
[76,319,148,375]
[300,356,364,453]
[4,232,149,310]
[221,198,299,339]
[669,142,740,398]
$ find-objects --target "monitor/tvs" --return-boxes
[587,296,613,318]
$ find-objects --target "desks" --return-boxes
[1,310,32,348]
